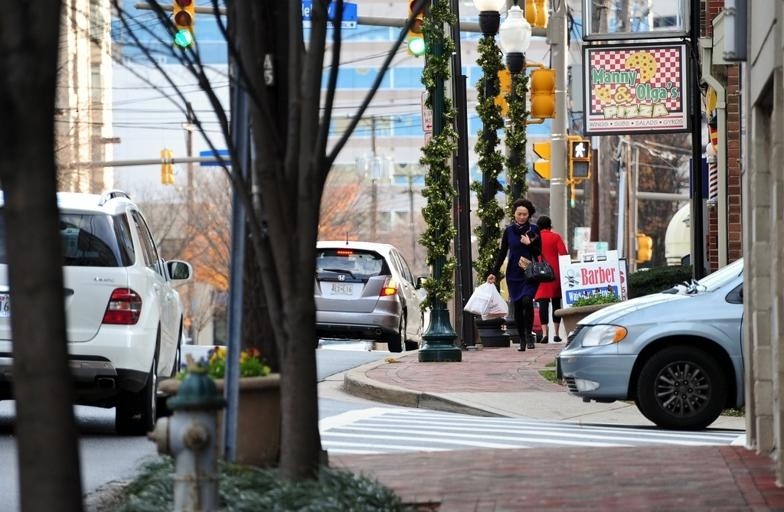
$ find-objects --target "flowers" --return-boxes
[185,347,271,377]
[574,284,622,305]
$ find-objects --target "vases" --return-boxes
[554,305,611,338]
[160,373,282,467]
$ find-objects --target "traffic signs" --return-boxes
[421,91,432,131]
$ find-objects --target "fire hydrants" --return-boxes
[148,362,227,512]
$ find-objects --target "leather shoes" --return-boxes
[518,337,526,350]
[527,334,533,350]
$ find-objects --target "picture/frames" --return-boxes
[580,41,690,135]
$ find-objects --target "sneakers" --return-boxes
[554,335,562,341]
[539,336,547,343]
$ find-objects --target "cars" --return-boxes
[0,190,192,436]
[313,241,427,352]
[555,257,743,430]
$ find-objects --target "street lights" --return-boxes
[473,0,532,347]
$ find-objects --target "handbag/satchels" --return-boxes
[525,262,555,282]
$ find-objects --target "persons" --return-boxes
[485,199,542,352]
[532,216,568,344]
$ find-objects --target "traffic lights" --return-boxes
[407,0,426,55]
[173,0,195,48]
[569,135,593,180]
